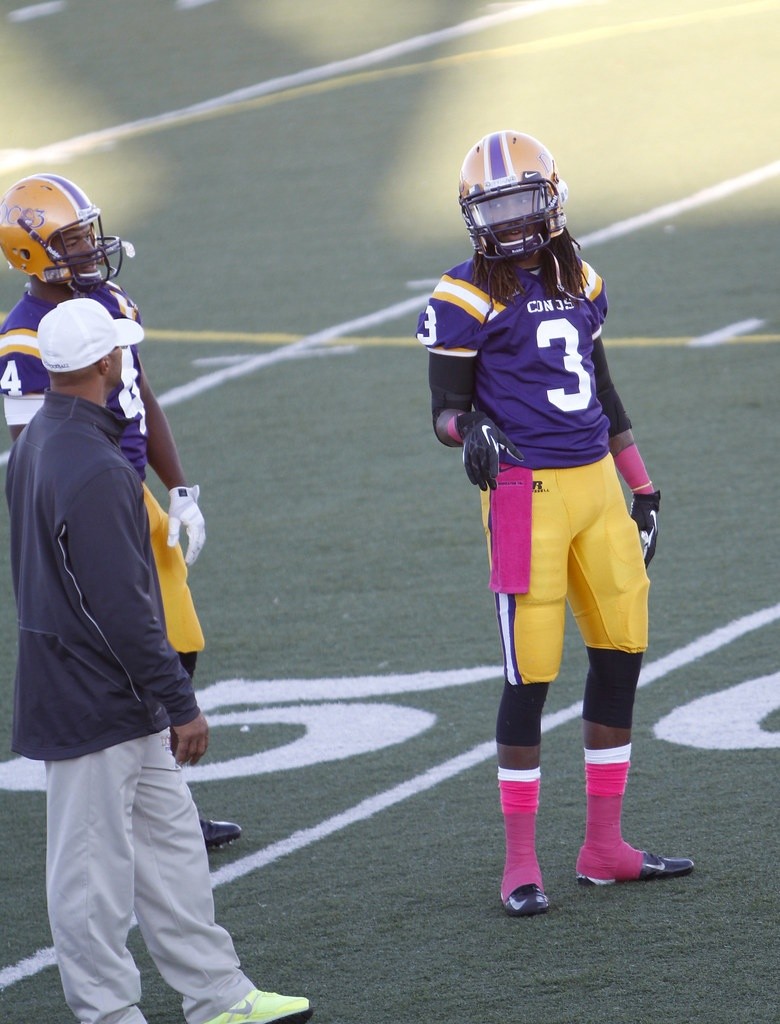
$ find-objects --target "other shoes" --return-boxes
[202,820,242,850]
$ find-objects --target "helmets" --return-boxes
[458,131,570,257]
[0,175,107,292]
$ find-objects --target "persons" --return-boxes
[415,129,695,914]
[6,298,313,1024]
[0,174,243,848]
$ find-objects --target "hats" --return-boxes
[38,298,145,372]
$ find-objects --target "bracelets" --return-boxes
[447,414,463,443]
[631,480,652,491]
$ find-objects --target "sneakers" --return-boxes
[502,858,549,916]
[576,851,694,885]
[203,988,314,1024]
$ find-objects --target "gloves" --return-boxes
[167,484,206,566]
[456,412,524,491]
[630,489,663,566]
